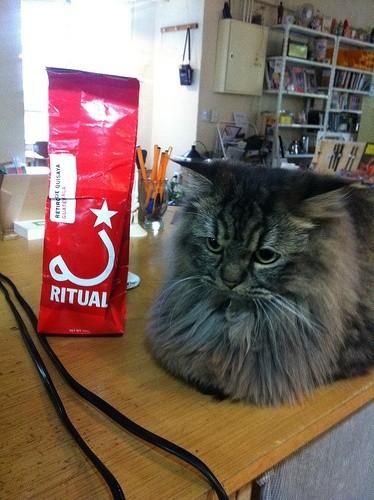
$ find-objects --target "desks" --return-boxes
[0,206,374,500]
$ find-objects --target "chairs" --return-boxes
[311,140,367,173]
[0,174,48,228]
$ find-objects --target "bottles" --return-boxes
[330,18,349,37]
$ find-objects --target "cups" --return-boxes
[139,178,168,218]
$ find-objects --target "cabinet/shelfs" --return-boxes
[257,24,374,169]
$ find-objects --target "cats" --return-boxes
[145,156,374,406]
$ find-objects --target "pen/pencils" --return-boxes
[135,145,173,218]
